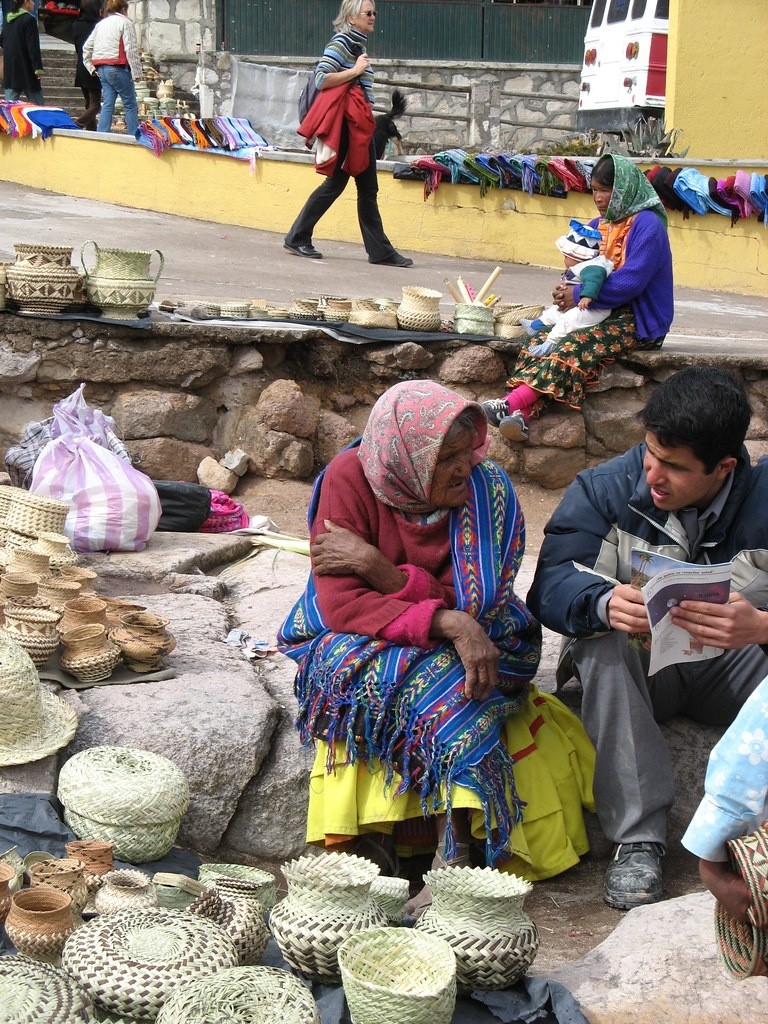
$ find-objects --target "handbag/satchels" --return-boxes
[299,71,324,123]
[3,383,249,553]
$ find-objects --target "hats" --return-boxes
[1,633,78,765]
[554,219,602,261]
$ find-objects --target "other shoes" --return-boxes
[528,340,556,356]
[353,833,401,876]
[402,840,472,916]
[520,317,537,336]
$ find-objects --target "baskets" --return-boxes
[350,310,399,328]
[494,303,544,339]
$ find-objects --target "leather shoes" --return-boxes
[604,835,666,908]
[284,238,323,257]
[372,249,413,267]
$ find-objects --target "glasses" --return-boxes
[359,10,378,17]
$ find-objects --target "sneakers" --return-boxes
[481,398,512,427]
[500,410,529,442]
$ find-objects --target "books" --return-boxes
[629,547,733,676]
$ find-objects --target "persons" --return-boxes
[481,153,674,441]
[520,220,614,358]
[681,676,768,923]
[0,0,45,104]
[73,0,104,132]
[284,0,413,267]
[82,0,142,135]
[525,369,768,911]
[277,380,596,927]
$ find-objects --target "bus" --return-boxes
[578,0,671,142]
